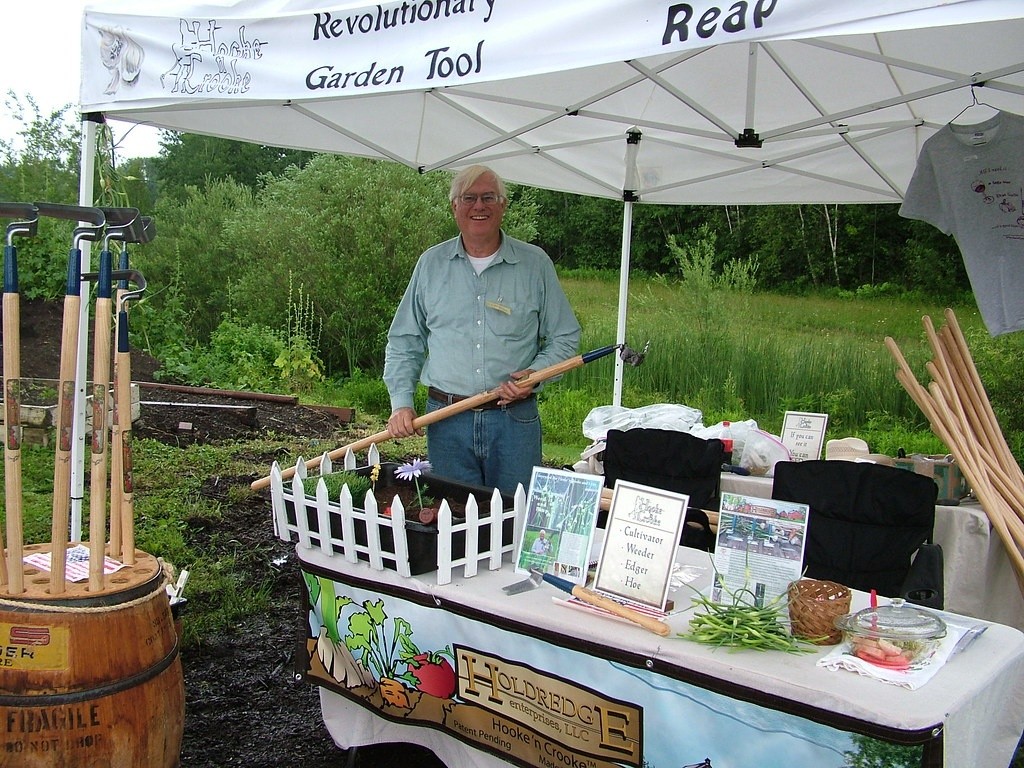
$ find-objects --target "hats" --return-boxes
[825,435,896,470]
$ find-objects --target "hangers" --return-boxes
[948,86,1000,123]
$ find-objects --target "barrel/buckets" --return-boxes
[2,539,186,767]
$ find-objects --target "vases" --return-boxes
[269,442,527,581]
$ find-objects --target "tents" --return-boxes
[67,0,1023,541]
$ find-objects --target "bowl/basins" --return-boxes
[833,599,947,670]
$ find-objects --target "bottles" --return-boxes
[719,422,733,470]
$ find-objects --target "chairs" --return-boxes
[596,428,723,555]
[771,460,944,611]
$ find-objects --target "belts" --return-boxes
[428,387,536,411]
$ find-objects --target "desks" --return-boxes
[295,527,1024,768]
[720,472,1024,633]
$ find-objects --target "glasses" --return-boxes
[454,192,503,206]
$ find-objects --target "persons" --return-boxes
[531,528,552,553]
[383,164,585,493]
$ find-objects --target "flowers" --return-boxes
[300,454,436,507]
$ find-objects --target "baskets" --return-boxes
[787,580,852,646]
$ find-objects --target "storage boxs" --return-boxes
[892,452,972,507]
